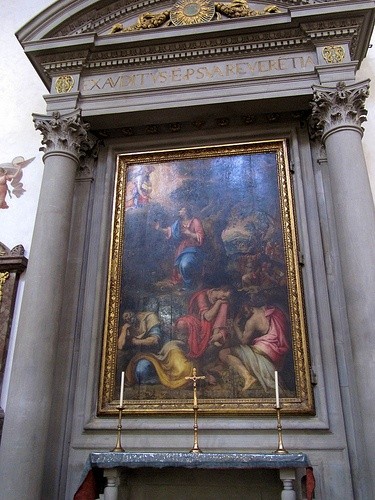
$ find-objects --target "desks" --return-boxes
[72,452,316,500]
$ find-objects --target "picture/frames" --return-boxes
[95,137,316,417]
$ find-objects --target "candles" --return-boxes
[119,372,125,408]
[275,370,280,408]
